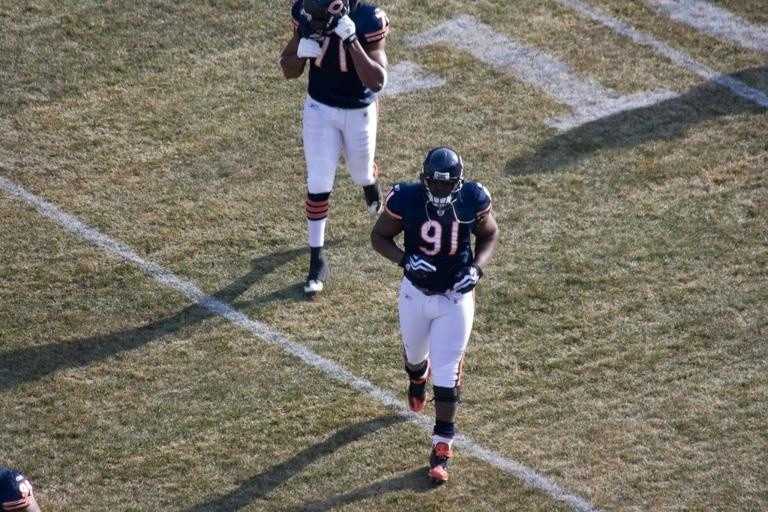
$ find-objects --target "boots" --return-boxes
[407,361,433,412]
[428,433,452,482]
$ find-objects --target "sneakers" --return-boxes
[303,258,329,295]
[362,180,382,217]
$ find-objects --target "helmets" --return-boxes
[302,0,350,34]
[421,147,463,209]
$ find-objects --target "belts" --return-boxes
[411,281,452,300]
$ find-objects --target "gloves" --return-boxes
[452,263,483,295]
[401,254,440,289]
[296,36,321,59]
[336,15,358,46]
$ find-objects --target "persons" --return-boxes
[278,0,390,297]
[369,146,499,484]
